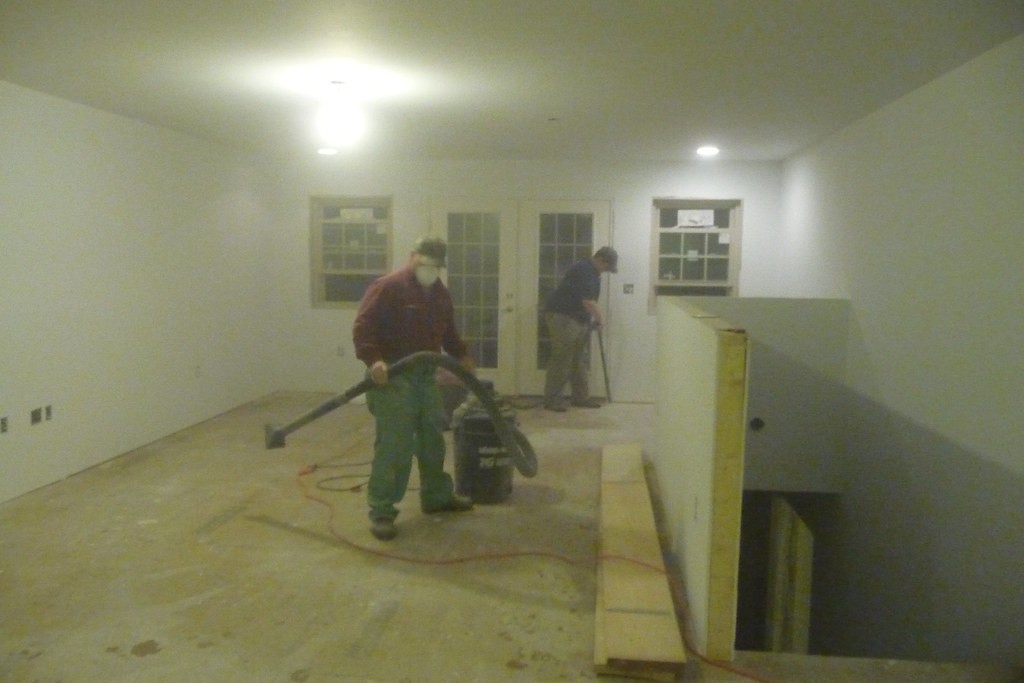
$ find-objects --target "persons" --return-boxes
[543,246,618,412]
[351,235,474,541]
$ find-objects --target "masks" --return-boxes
[415,265,440,286]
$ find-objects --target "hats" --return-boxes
[415,235,447,268]
[598,247,618,274]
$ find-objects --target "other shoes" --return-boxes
[573,399,600,408]
[372,518,394,539]
[422,494,473,513]
[545,405,567,413]
